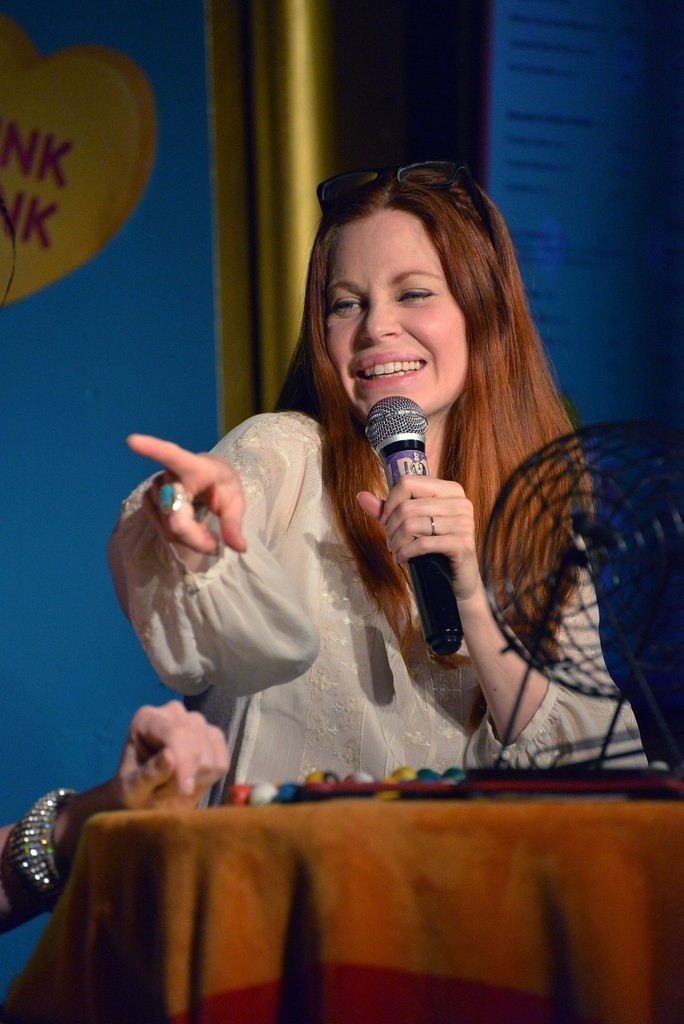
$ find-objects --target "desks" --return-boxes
[0,795,684,1023]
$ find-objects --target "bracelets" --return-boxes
[8,787,82,915]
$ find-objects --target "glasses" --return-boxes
[317,156,499,255]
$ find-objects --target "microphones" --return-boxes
[365,396,467,655]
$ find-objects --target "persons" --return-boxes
[0,699,230,932]
[108,158,646,789]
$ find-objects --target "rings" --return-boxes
[430,517,435,536]
[159,483,194,516]
